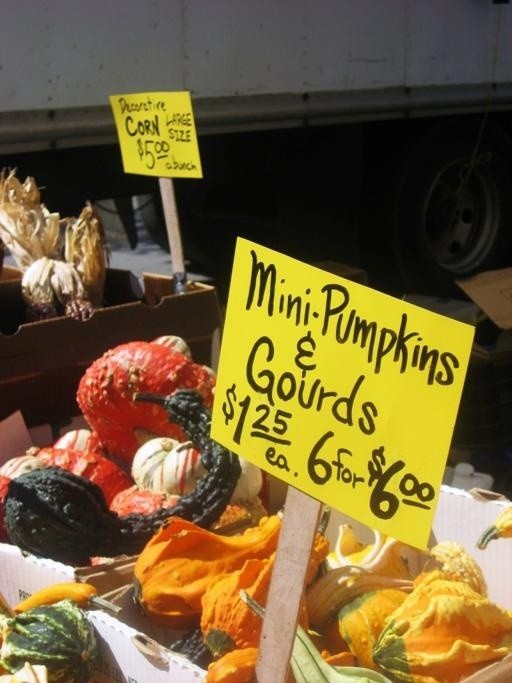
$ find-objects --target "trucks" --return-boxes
[2,0,512,291]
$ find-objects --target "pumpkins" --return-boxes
[134,502,512,683]
[0,582,123,683]
[1,336,263,566]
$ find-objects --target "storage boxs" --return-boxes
[1,268,223,441]
